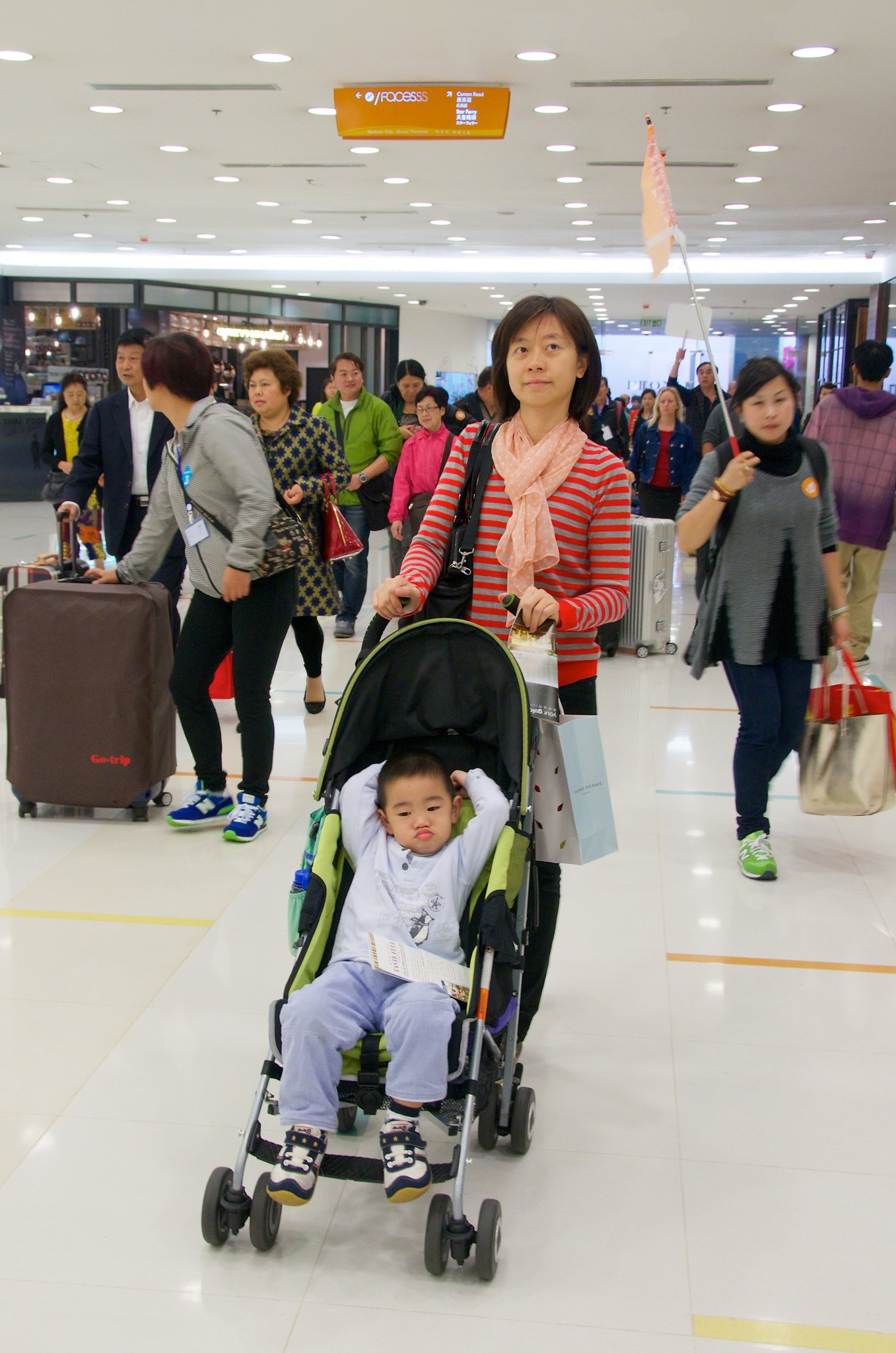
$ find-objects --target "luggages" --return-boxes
[0,510,179,823]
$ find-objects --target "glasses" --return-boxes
[414,406,440,414]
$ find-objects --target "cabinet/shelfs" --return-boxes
[168,312,309,348]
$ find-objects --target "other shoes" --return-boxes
[237,723,241,733]
[304,677,326,714]
[851,653,869,667]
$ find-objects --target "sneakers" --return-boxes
[737,832,778,880]
[223,792,267,842]
[334,619,355,636]
[380,1116,433,1204]
[165,779,234,826]
[267,1120,327,1205]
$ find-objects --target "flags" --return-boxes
[641,127,676,276]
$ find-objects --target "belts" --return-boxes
[129,494,149,507]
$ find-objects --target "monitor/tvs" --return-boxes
[41,383,62,399]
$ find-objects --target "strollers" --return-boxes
[201,590,554,1284]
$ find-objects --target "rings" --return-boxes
[743,462,750,472]
[741,452,746,459]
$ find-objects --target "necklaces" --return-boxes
[404,405,416,414]
[64,409,85,420]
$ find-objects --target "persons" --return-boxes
[41,373,106,572]
[56,332,297,842]
[267,755,511,1206]
[802,340,896,674]
[229,349,345,735]
[313,345,839,659]
[374,296,630,1058]
[677,355,846,882]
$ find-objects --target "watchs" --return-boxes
[358,472,367,484]
[709,482,732,503]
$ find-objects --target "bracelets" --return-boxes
[827,605,850,618]
[713,477,734,495]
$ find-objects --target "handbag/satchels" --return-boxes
[41,471,71,504]
[407,491,433,538]
[357,476,392,531]
[793,639,896,816]
[413,418,504,626]
[209,646,234,699]
[533,701,617,865]
[251,517,313,579]
[320,472,364,563]
